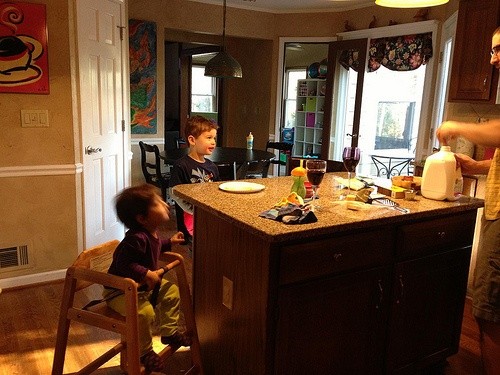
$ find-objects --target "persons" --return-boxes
[435,26,500,349]
[102,184,193,372]
[171,114,222,237]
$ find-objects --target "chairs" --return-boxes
[139,140,294,203]
[51,239,205,375]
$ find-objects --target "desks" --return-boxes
[203,146,276,181]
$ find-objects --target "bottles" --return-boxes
[421,145,461,200]
[246,131,254,150]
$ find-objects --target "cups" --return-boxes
[404,190,419,199]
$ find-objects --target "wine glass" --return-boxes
[343,148,360,195]
[306,159,327,207]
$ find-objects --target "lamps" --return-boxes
[286,43,304,52]
[204,0,242,79]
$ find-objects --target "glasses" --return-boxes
[490,45,500,57]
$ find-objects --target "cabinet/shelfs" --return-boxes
[292,78,327,156]
[448,0,500,106]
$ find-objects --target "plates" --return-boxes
[219,182,265,193]
[393,176,422,188]
